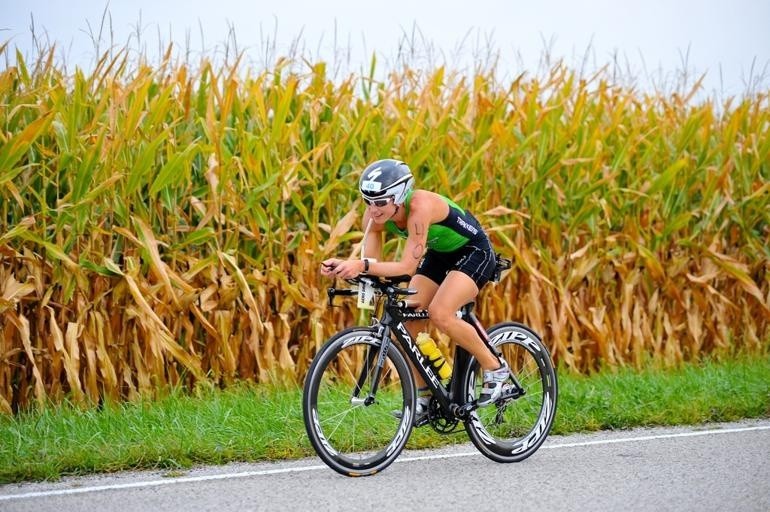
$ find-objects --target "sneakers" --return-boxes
[392,396,431,421]
[477,357,510,406]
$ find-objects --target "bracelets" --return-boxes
[359,259,369,276]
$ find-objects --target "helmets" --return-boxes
[358,159,414,204]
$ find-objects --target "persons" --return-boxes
[320,158,512,424]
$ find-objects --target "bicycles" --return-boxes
[302,273,557,477]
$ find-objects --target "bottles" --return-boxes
[416,332,452,380]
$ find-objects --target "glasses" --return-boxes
[362,195,392,207]
